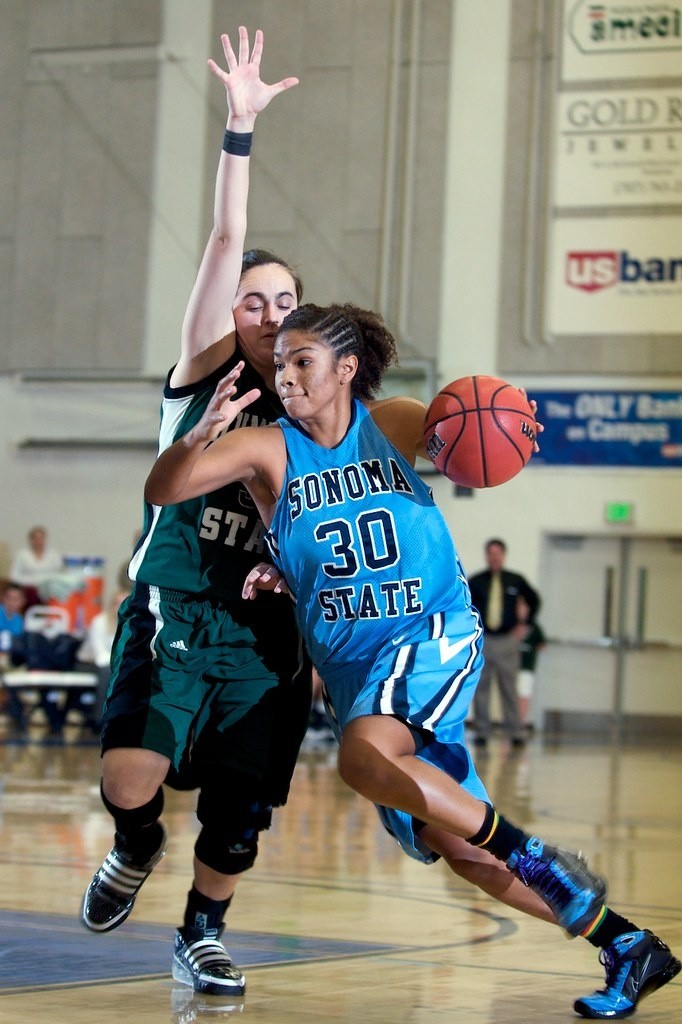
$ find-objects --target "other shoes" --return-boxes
[512,739,523,745]
[473,738,485,745]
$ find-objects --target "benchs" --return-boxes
[2,670,98,737]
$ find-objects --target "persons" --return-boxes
[466,538,541,749]
[144,303,682,1023]
[77,592,131,740]
[502,590,548,731]
[12,527,66,603]
[89,24,317,997]
[0,585,27,683]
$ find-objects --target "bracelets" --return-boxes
[222,129,253,156]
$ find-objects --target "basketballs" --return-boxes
[424,374,537,489]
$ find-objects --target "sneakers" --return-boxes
[80,823,167,933]
[574,929,682,1019]
[506,836,605,938]
[171,926,246,996]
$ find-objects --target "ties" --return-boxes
[486,573,502,631]
